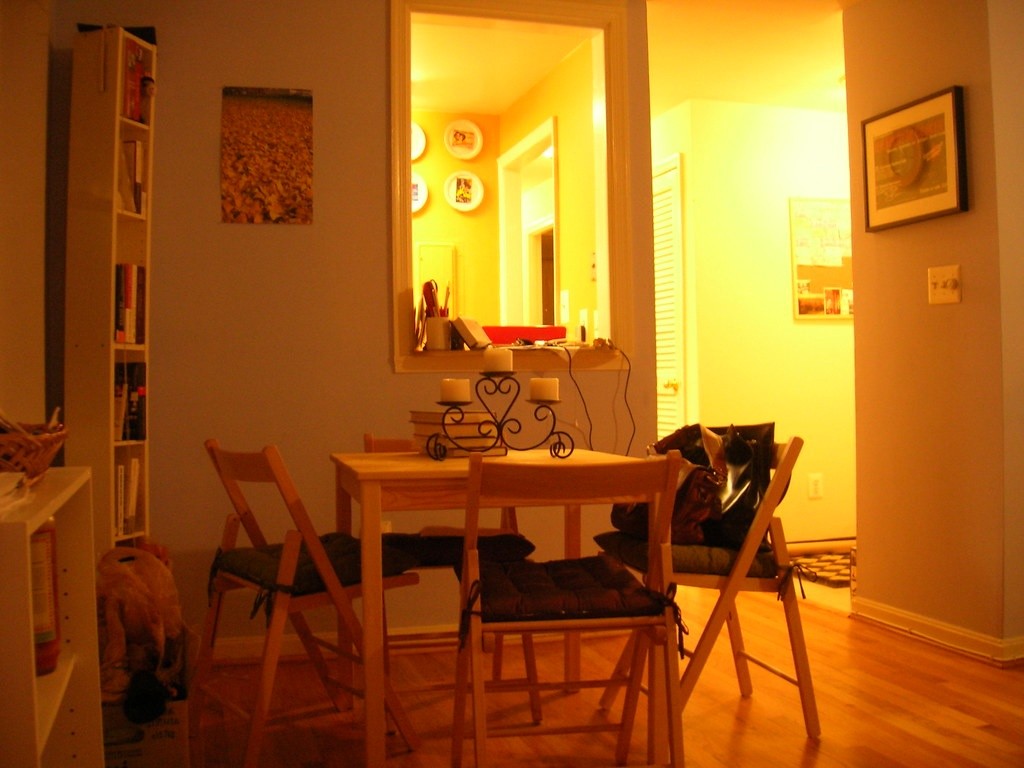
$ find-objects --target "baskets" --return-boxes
[0,422,69,480]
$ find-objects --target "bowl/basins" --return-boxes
[481,324,565,344]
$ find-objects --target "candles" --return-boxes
[440,378,471,401]
[483,344,513,372]
[530,375,560,401]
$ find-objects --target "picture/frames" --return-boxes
[861,85,968,233]
[411,121,428,161]
[411,172,429,213]
[444,170,486,213]
[442,118,484,161]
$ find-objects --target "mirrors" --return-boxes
[390,0,636,374]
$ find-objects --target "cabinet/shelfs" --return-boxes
[64,27,160,567]
[0,465,105,768]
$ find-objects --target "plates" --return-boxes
[412,173,428,214]
[411,120,426,161]
[443,119,484,161]
[444,170,485,212]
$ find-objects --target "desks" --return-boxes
[329,448,672,768]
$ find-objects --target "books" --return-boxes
[113,263,147,538]
[121,140,144,215]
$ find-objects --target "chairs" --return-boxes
[191,436,822,768]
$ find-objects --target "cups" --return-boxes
[424,317,452,351]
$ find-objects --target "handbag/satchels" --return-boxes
[611,422,776,546]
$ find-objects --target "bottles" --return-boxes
[798,279,850,314]
